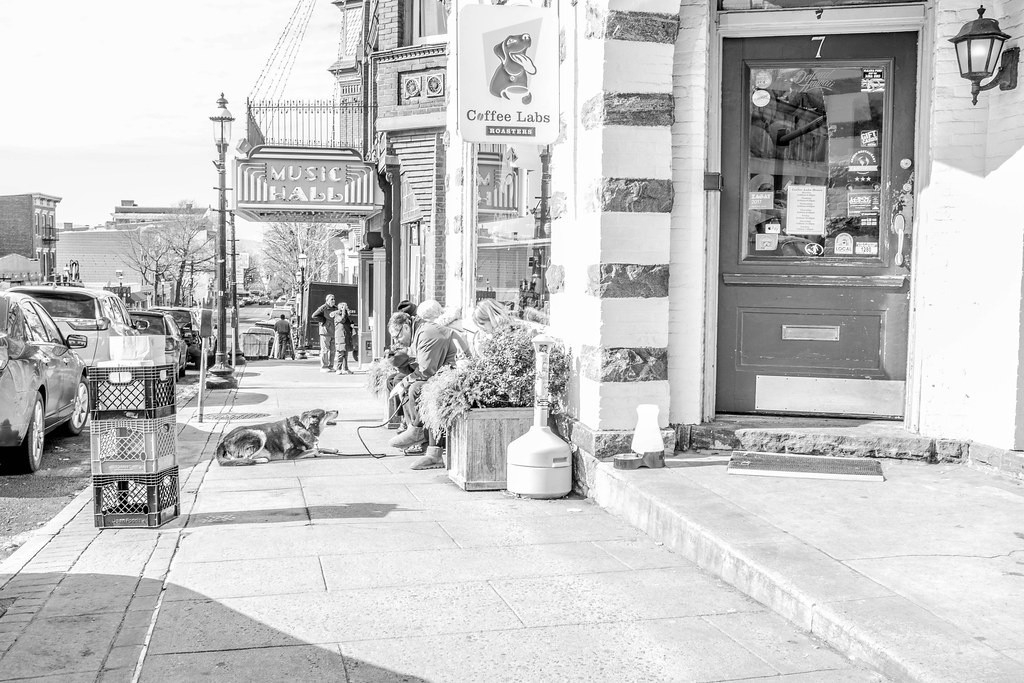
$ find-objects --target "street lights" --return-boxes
[203,91,238,389]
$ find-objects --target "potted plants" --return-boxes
[413,320,571,492]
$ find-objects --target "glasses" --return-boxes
[392,324,403,342]
[331,299,335,300]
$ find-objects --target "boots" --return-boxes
[389,425,424,448]
[410,447,444,470]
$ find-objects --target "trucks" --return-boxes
[299,282,359,362]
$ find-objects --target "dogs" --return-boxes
[216,408,339,467]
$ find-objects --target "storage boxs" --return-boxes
[85,363,181,529]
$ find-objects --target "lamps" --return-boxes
[948,5,1021,107]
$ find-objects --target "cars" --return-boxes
[223,284,300,351]
[149,307,216,371]
[9,285,150,370]
[128,310,189,382]
[0,290,89,472]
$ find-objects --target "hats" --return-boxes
[397,299,417,317]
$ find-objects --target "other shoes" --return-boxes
[320,367,336,373]
[336,369,354,375]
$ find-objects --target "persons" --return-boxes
[311,293,340,373]
[330,301,355,375]
[383,297,529,472]
[274,314,290,359]
